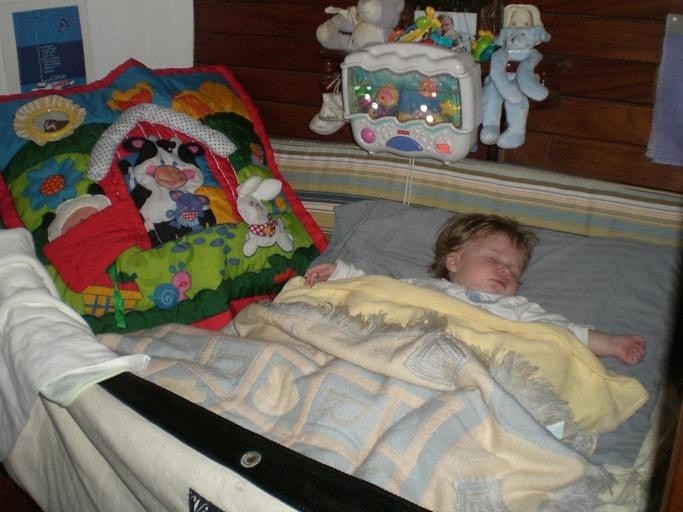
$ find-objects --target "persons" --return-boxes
[305,212,646,366]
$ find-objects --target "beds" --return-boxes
[0,137,683,505]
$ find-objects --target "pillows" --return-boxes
[0,58,330,336]
[307,198,680,469]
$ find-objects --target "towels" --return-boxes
[0,227,153,465]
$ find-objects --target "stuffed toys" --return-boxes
[309,0,554,168]
[309,0,553,168]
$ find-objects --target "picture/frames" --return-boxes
[1,0,93,96]
